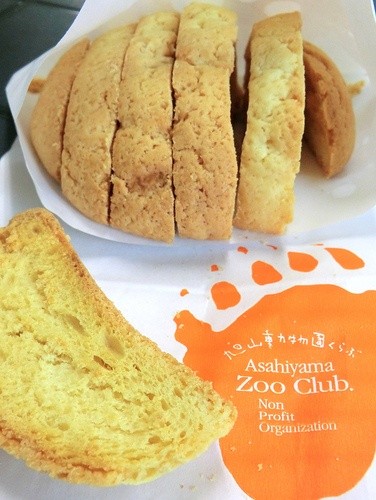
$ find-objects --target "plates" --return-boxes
[7,0,374,249]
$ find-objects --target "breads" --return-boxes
[0,2,355,488]
